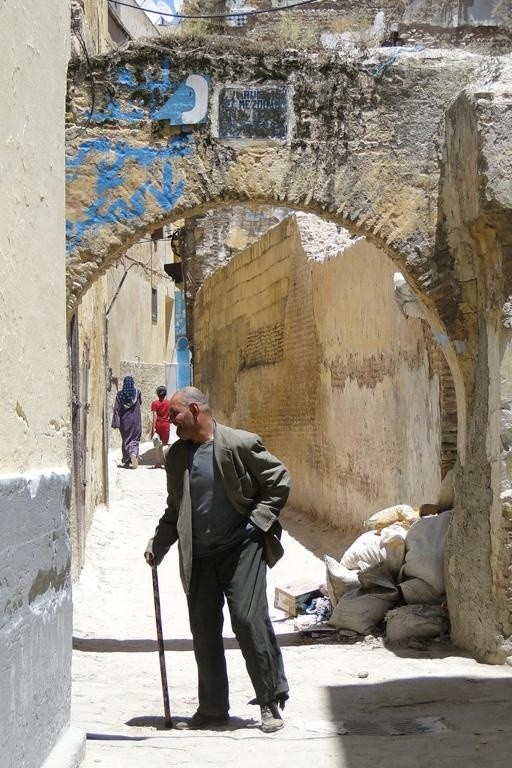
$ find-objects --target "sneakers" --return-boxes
[122,454,165,468]
[175,708,232,731]
[260,701,286,733]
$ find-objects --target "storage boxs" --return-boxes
[274,584,321,618]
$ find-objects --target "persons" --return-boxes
[144,387,290,733]
[111,376,142,469]
[150,385,172,468]
